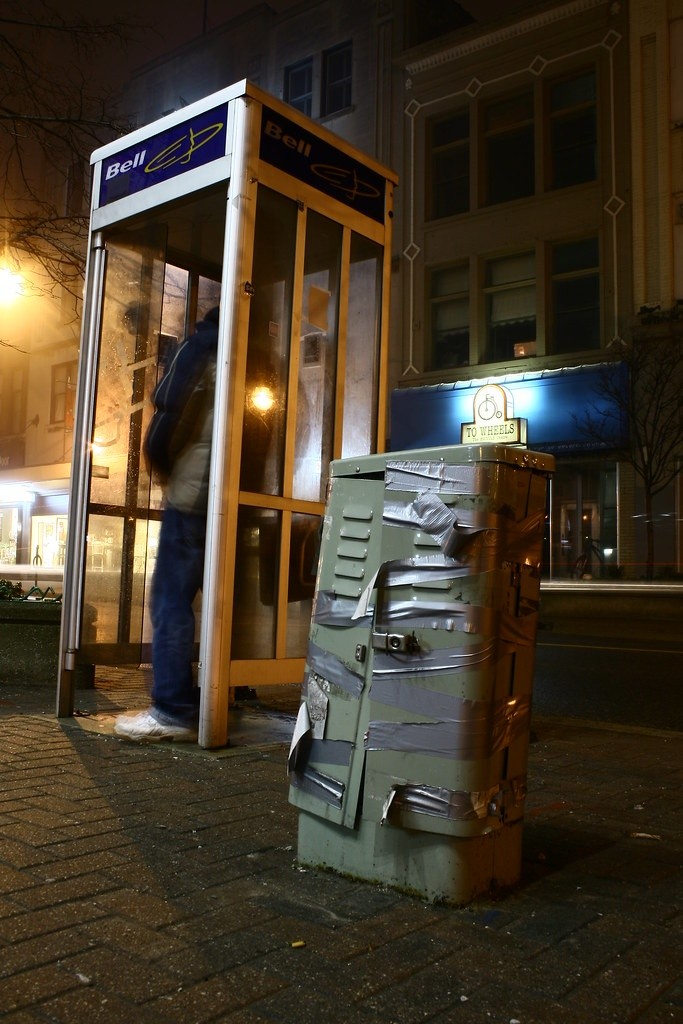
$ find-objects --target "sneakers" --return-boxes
[114,710,199,744]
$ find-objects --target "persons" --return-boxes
[114,279,312,746]
